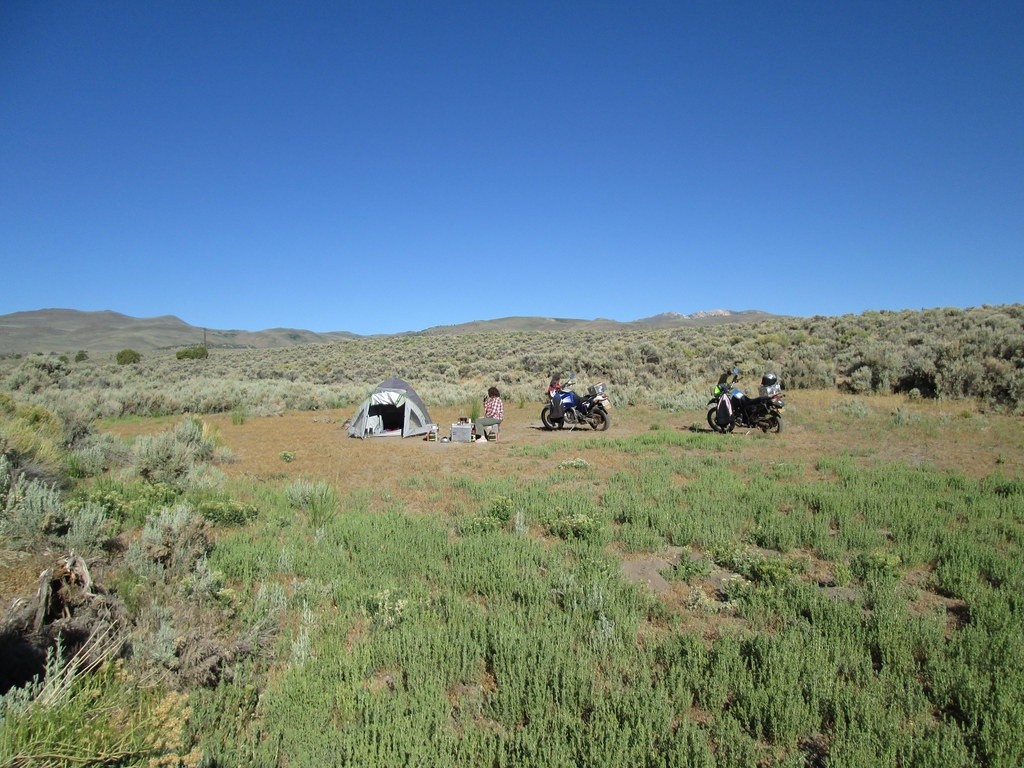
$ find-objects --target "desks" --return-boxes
[451,422,476,442]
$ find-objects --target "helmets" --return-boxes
[760,371,777,386]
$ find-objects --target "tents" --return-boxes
[345,378,439,440]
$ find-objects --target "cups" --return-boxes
[429,432,435,441]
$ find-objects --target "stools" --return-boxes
[426,430,438,442]
[486,423,499,442]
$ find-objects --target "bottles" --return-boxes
[472,425,476,432]
[471,431,476,442]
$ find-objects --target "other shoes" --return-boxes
[475,438,487,443]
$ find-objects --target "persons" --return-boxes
[475,387,504,443]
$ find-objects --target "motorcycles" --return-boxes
[707,367,785,436]
[541,372,611,433]
